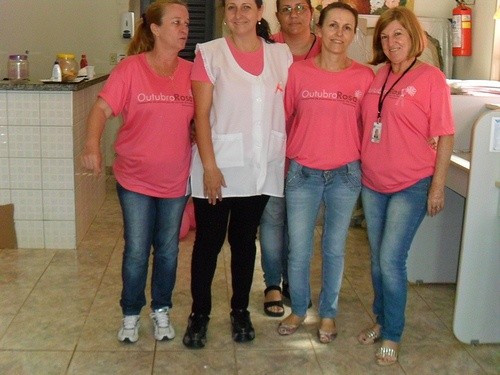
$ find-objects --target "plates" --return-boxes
[41,78,84,83]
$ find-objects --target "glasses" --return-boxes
[278,4,309,15]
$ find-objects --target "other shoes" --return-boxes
[183,311,211,349]
[230,309,256,343]
[318,317,338,343]
[278,312,307,335]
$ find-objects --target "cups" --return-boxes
[86,65,94,79]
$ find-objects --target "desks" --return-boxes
[407,94,500,345]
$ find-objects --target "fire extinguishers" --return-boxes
[452,0,472,56]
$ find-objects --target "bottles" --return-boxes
[52,62,62,81]
[80,55,88,69]
[7,55,29,81]
[56,53,79,81]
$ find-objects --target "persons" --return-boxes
[277,2,375,342]
[360,6,455,365]
[264,0,323,316]
[183,0,292,349]
[374,129,378,138]
[80,0,195,343]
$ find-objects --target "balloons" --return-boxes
[179,202,196,240]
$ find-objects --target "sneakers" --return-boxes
[149,306,175,342]
[117,314,145,343]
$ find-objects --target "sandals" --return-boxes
[264,285,285,317]
[376,343,401,366]
[281,282,312,308]
[357,326,380,345]
